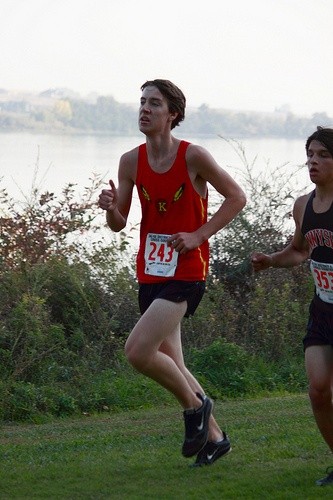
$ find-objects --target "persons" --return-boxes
[99,79,247,468]
[250,126,333,487]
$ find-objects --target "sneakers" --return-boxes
[181,392,213,459]
[315,471,333,487]
[188,431,232,468]
